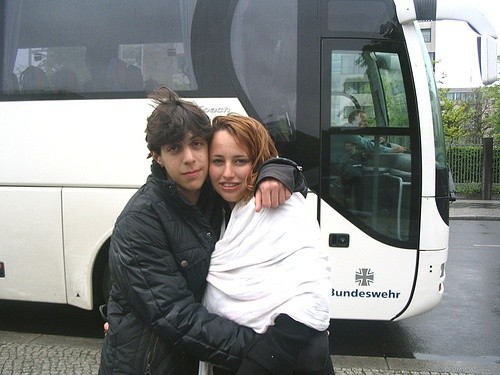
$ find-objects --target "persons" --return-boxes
[98,86,308,374]
[190,110,337,375]
[331,108,407,213]
[1,50,160,91]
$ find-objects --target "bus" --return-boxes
[1,0,500,341]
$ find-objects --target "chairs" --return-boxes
[0,57,164,92]
